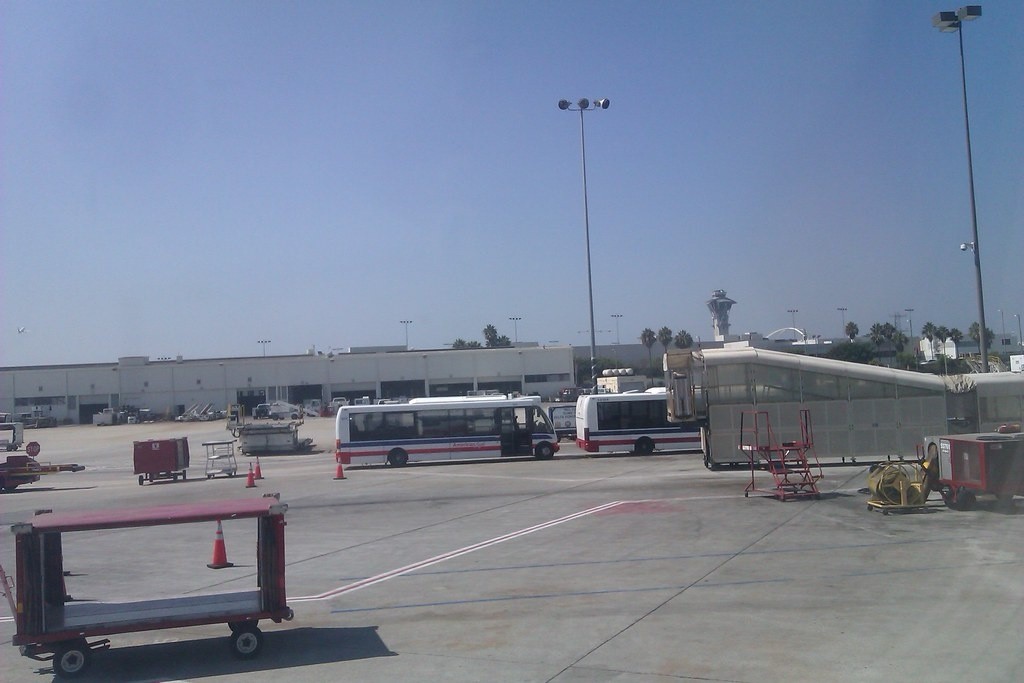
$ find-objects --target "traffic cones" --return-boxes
[246,456,264,488]
[333,456,347,480]
[206,518,234,569]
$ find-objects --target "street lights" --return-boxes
[837,308,849,335]
[257,339,271,357]
[399,320,414,349]
[786,310,799,338]
[996,309,1007,365]
[508,317,522,343]
[558,97,611,393]
[903,308,916,337]
[610,314,623,343]
[930,4,989,373]
[1013,314,1022,355]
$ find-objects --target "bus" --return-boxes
[336,394,560,460]
[575,385,784,455]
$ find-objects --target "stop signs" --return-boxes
[26,442,40,456]
